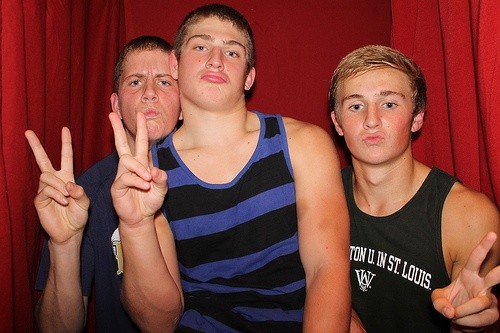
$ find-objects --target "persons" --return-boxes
[328,43,500,333]
[107,4,352,333]
[22,35,185,333]
